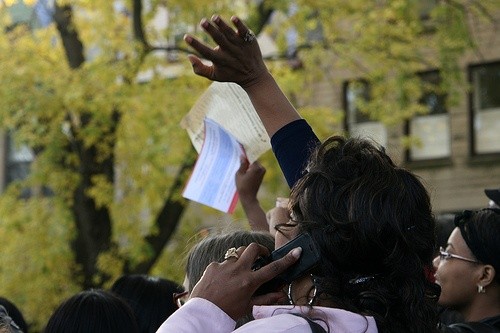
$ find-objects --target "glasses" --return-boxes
[173,291,188,309]
[440,246,484,266]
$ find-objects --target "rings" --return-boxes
[223,247,239,260]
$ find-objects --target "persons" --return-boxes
[0,12,500,332]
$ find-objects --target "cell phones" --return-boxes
[253,233,320,289]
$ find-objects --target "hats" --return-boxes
[484,190,500,206]
